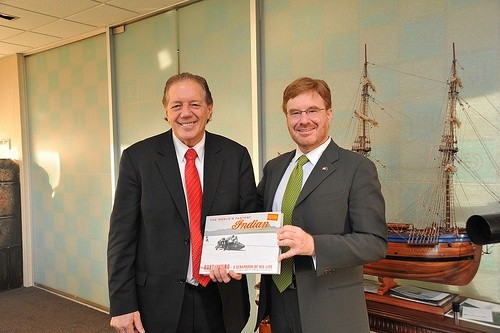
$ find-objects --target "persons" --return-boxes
[256,76,387,333]
[107,71,261,333]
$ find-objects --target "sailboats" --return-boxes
[330,42,500,286]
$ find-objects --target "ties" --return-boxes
[271,155,310,293]
[182,148,212,288]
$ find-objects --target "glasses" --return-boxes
[287,108,328,117]
